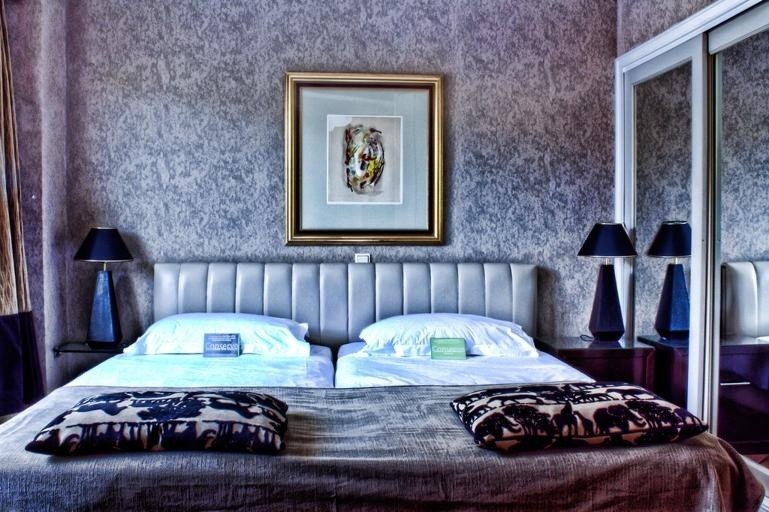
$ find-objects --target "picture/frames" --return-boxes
[283,71,446,246]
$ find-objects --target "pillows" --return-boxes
[124,311,309,360]
[354,312,539,362]
[22,391,289,461]
[451,381,708,456]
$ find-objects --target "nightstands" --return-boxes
[541,337,654,392]
[636,334,769,452]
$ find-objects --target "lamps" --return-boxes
[72,227,135,349]
[643,219,692,340]
[576,222,638,342]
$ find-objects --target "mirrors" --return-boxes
[705,0,769,507]
[613,22,708,440]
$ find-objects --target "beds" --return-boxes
[0,342,333,512]
[721,260,769,349]
[334,342,763,512]
